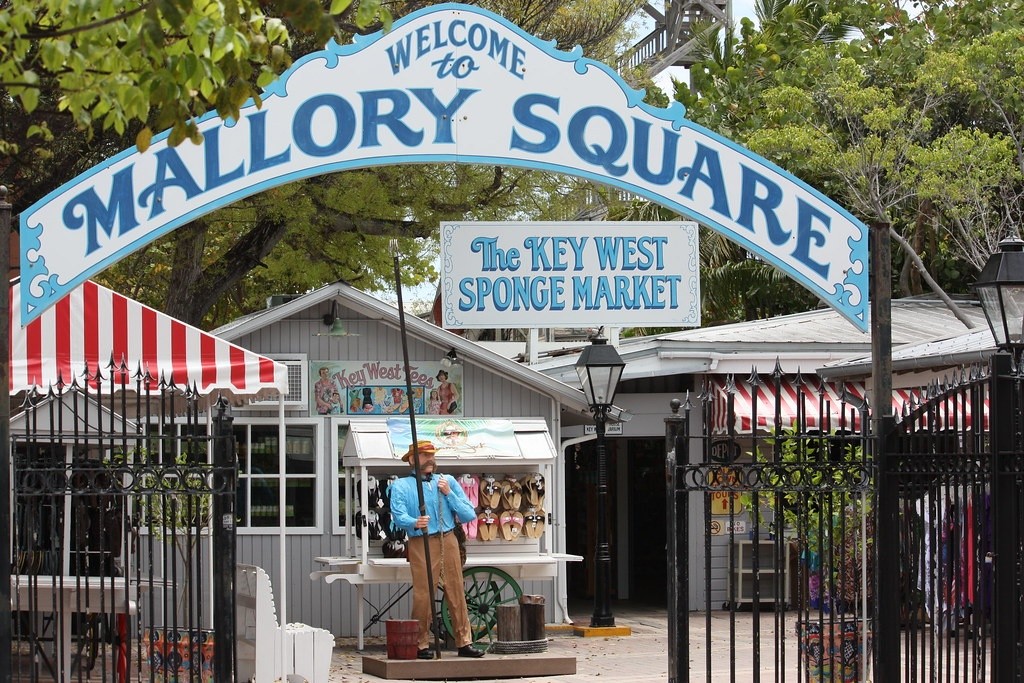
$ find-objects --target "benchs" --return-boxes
[237,562,336,683]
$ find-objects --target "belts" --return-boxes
[411,529,453,538]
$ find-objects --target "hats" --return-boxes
[402,441,435,462]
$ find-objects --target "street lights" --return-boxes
[975,226,1024,683]
[572,327,627,628]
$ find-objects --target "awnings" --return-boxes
[704,376,989,432]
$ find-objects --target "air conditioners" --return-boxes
[248,361,308,406]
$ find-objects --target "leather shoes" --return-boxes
[458,644,485,657]
[416,648,434,659]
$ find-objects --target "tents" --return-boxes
[8,274,290,683]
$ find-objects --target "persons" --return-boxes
[390,442,484,659]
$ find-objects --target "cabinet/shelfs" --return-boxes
[238,425,313,526]
[727,539,790,603]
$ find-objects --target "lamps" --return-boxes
[312,299,362,339]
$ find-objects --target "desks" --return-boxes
[309,553,584,651]
[10,575,129,682]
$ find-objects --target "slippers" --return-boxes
[354,474,547,542]
